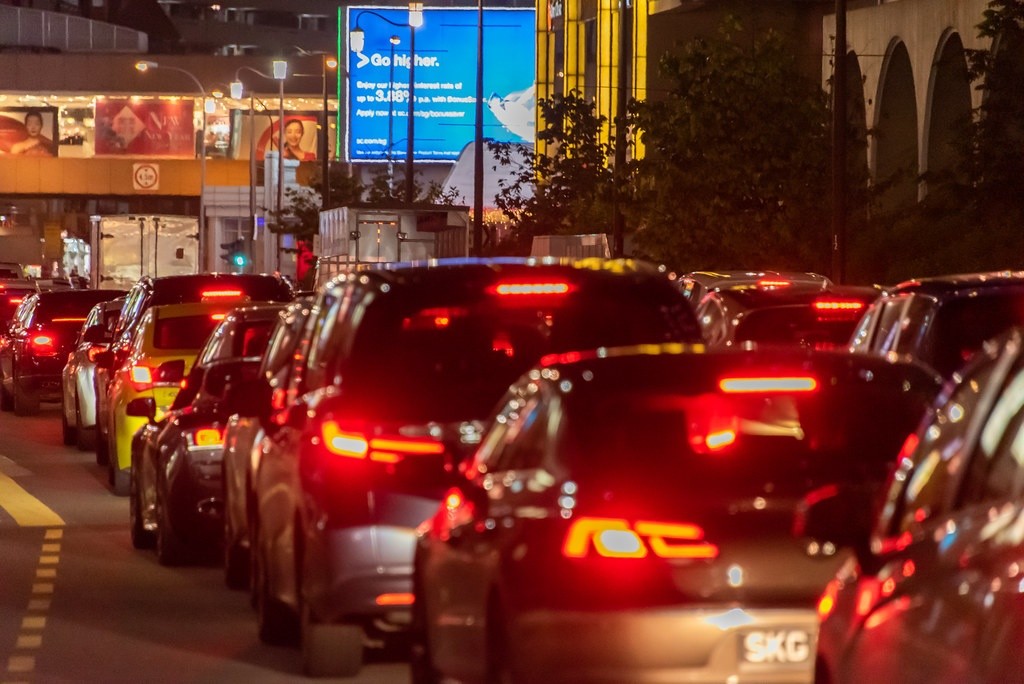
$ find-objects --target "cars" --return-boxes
[0,292,137,416]
[850,268,1023,380]
[81,272,296,471]
[386,342,943,684]
[679,269,832,309]
[220,286,310,587]
[695,286,877,344]
[203,256,703,676]
[0,278,43,330]
[90,301,243,494]
[61,299,125,452]
[797,325,1023,682]
[155,302,291,382]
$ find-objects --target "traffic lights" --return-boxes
[221,241,244,264]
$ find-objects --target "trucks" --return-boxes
[312,203,472,287]
[89,213,208,292]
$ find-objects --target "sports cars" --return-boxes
[125,357,260,567]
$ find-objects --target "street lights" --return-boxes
[349,10,415,205]
[135,61,208,275]
[212,84,274,269]
[228,65,285,272]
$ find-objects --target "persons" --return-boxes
[11,112,54,156]
[283,120,315,160]
